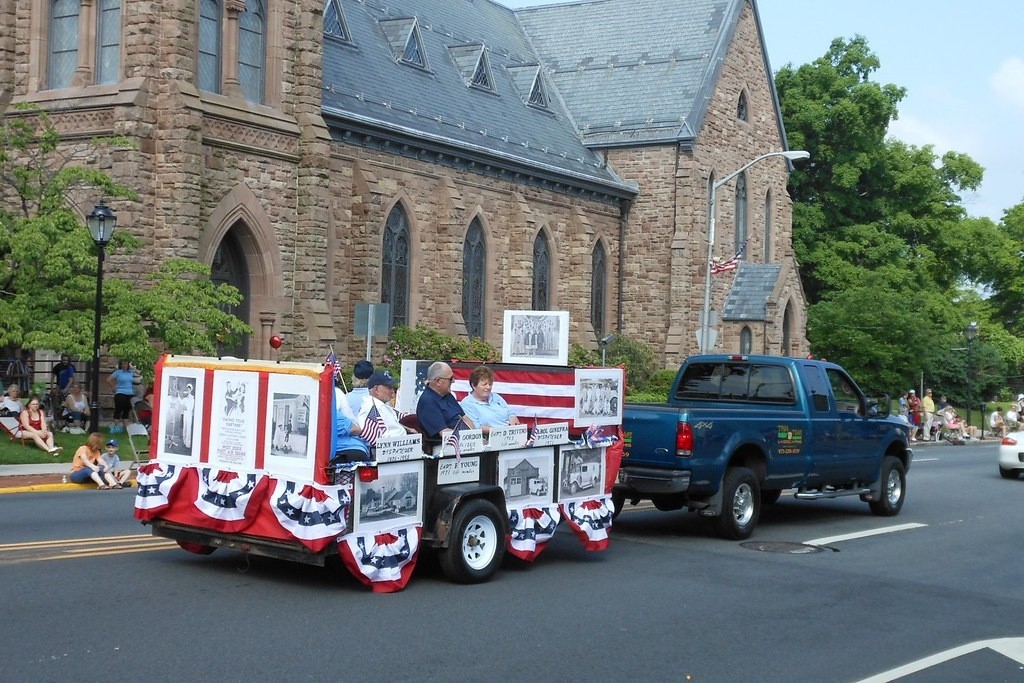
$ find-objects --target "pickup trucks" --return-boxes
[611,353,916,539]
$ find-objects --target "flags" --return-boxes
[447,426,461,463]
[360,404,387,446]
[326,351,341,384]
[527,415,538,448]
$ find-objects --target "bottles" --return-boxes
[62,475,68,485]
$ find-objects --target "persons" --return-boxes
[331,360,418,463]
[107,359,142,432]
[180,383,194,448]
[8,348,29,397]
[899,388,1024,445]
[70,432,123,490]
[0,384,23,414]
[101,439,132,488]
[18,396,63,456]
[140,379,154,424]
[458,366,520,434]
[416,362,476,438]
[0,381,4,402]
[51,354,74,414]
[61,381,91,433]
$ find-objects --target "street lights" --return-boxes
[964,325,976,426]
[85,199,118,431]
[700,150,810,355]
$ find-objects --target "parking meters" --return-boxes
[980,403,986,439]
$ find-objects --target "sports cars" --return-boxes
[999,430,1024,479]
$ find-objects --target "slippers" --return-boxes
[108,483,123,489]
[97,484,108,490]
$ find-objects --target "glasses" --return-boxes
[439,375,453,383]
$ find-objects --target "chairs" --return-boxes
[126,423,150,472]
[0,416,49,449]
[720,371,745,395]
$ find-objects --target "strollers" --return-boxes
[132,398,152,446]
[917,412,943,441]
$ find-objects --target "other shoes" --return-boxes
[910,434,981,444]
[120,481,132,487]
[48,446,62,456]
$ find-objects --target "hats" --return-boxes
[353,360,374,379]
[909,389,915,394]
[367,370,399,388]
[925,389,932,393]
[105,439,119,447]
[997,407,1002,411]
